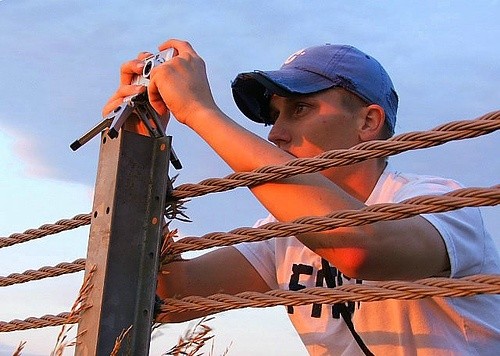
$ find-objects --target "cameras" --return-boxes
[129,47,179,87]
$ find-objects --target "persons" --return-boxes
[103,35,500,356]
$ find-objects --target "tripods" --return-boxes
[70,92,183,171]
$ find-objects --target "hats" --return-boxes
[230,43,399,135]
